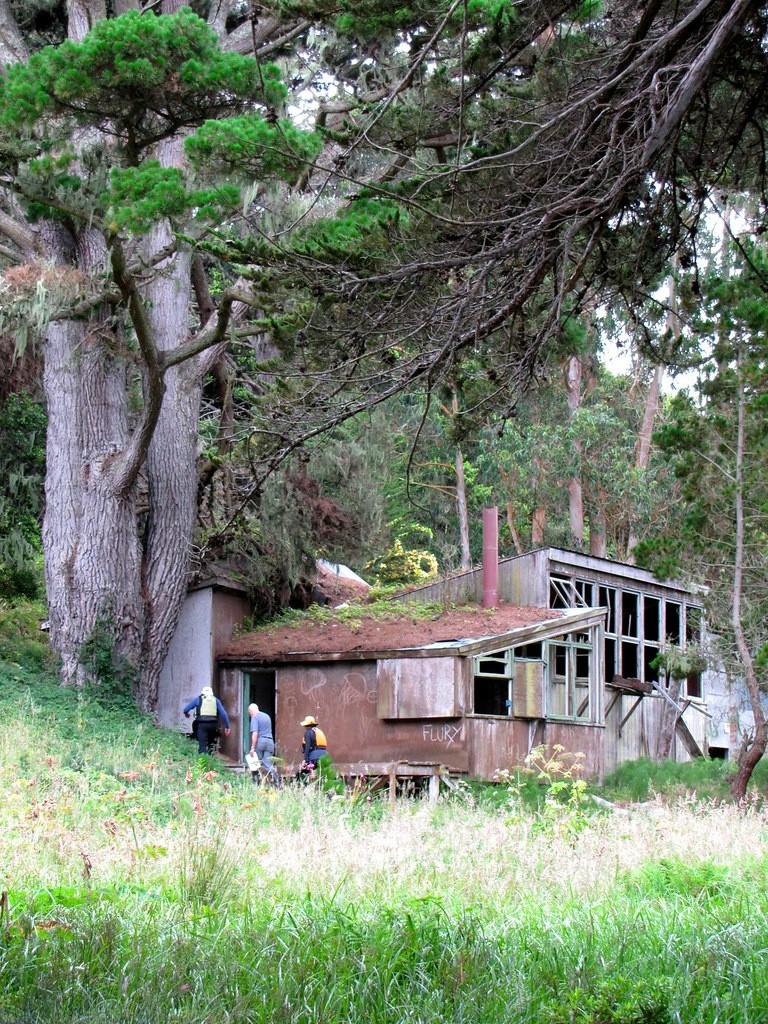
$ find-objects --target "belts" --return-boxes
[262,736,273,740]
[309,746,327,752]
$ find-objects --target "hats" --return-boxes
[300,716,318,727]
[201,686,213,696]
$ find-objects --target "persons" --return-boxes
[183,687,231,756]
[247,703,281,791]
[296,715,327,790]
